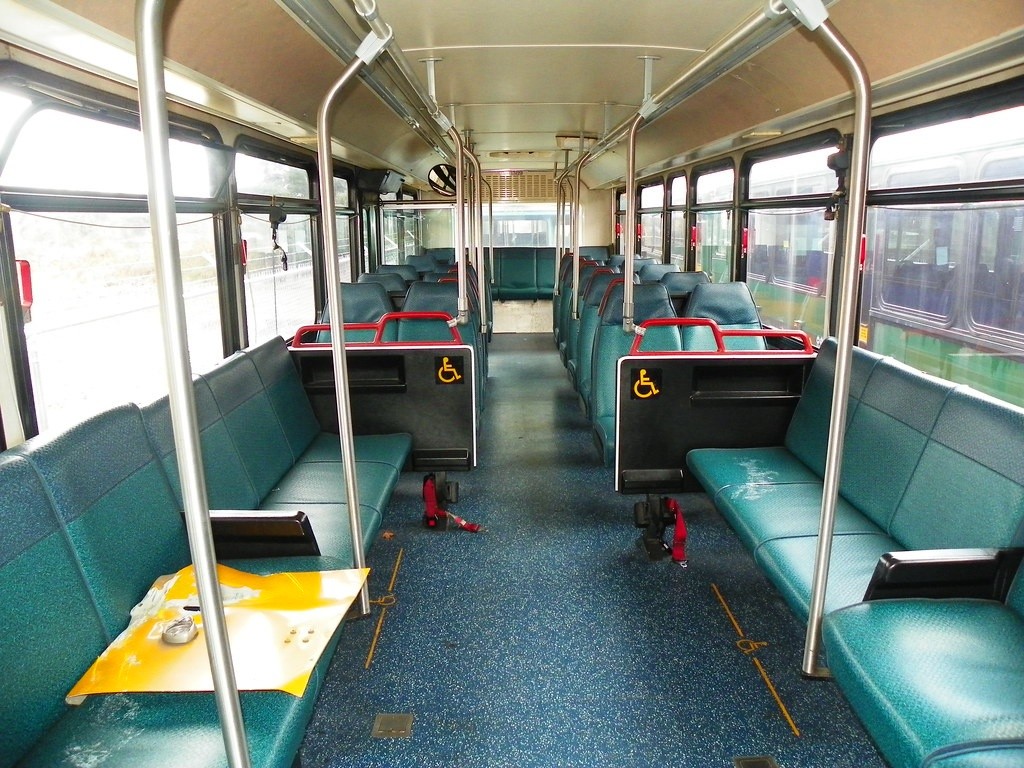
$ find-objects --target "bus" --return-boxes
[642,137,1022,405]
[477,211,571,248]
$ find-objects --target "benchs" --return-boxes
[1,247,1024,767]
